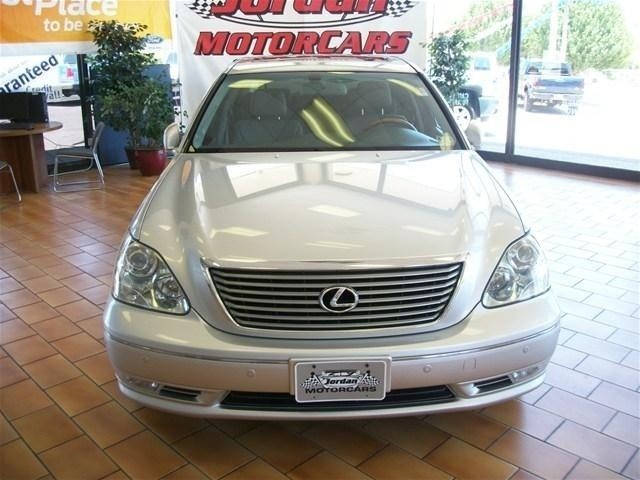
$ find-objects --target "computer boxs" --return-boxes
[29,92,49,122]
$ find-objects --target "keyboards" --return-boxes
[0,122,32,128]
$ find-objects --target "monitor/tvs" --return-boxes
[0,93,31,122]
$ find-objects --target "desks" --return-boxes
[0,121,63,193]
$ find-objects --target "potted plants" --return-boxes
[84,19,188,176]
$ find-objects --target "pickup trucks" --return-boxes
[517,60,585,115]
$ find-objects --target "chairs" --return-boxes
[0,161,21,202]
[233,88,303,146]
[349,81,408,132]
[53,122,106,193]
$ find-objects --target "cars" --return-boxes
[430,79,482,131]
[104,55,561,421]
[1,56,80,102]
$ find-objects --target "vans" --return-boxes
[429,52,501,116]
